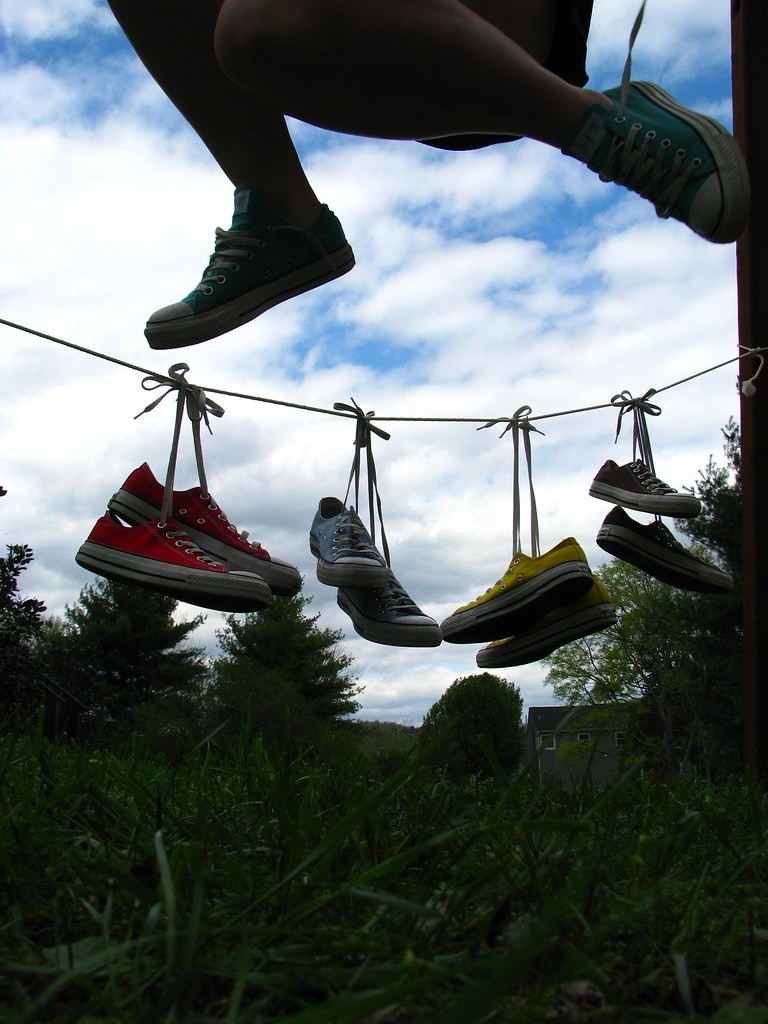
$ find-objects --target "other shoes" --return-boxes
[76,464,302,612]
[440,537,616,668]
[309,496,442,648]
[589,460,734,594]
[559,81,751,244]
[145,185,355,350]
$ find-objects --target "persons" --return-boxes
[105,1,750,349]
